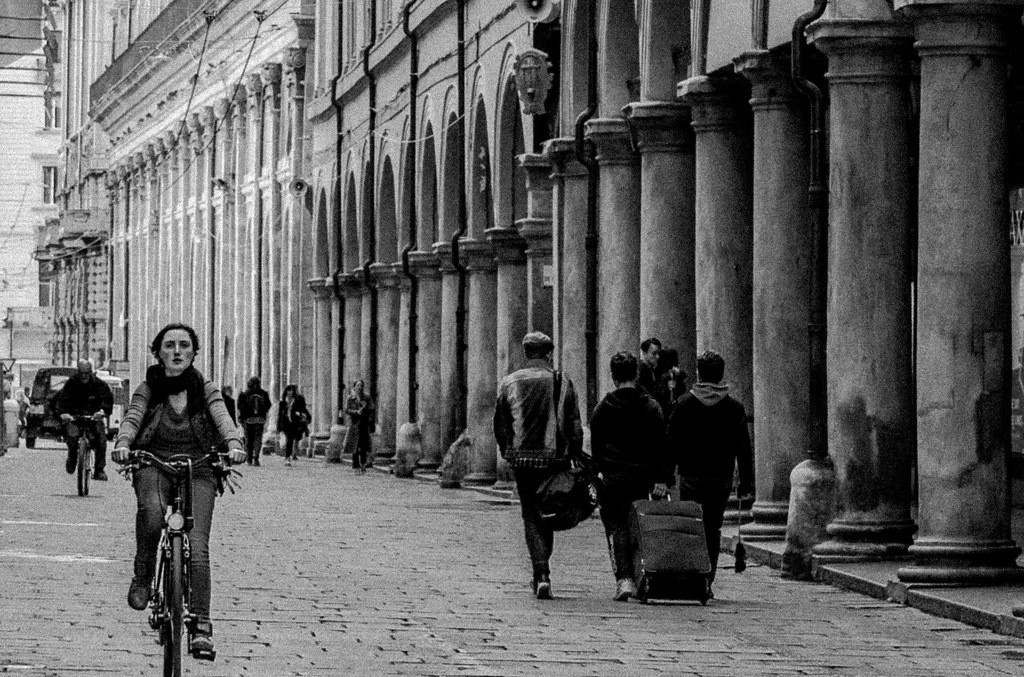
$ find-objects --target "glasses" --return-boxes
[77,371,91,376]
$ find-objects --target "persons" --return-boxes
[120,379,128,417]
[19,387,32,438]
[50,359,114,481]
[275,384,312,466]
[221,386,237,425]
[343,381,376,476]
[591,352,677,601]
[112,324,247,653]
[635,339,690,407]
[668,352,755,589]
[236,376,272,466]
[493,332,583,597]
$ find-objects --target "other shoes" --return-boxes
[247,458,253,466]
[353,468,360,476]
[192,633,214,649]
[613,578,633,601]
[284,457,291,466]
[532,574,556,600]
[128,578,150,610]
[254,458,260,466]
[360,465,366,474]
[66,455,77,474]
[94,470,108,481]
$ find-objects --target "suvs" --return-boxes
[24,366,81,449]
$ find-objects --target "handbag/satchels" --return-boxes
[531,448,608,531]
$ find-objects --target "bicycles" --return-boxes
[124,446,230,676]
[66,415,98,497]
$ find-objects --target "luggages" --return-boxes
[628,488,714,605]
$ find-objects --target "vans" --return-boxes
[97,375,129,441]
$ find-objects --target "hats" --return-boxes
[522,331,553,345]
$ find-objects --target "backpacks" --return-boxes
[245,394,267,424]
